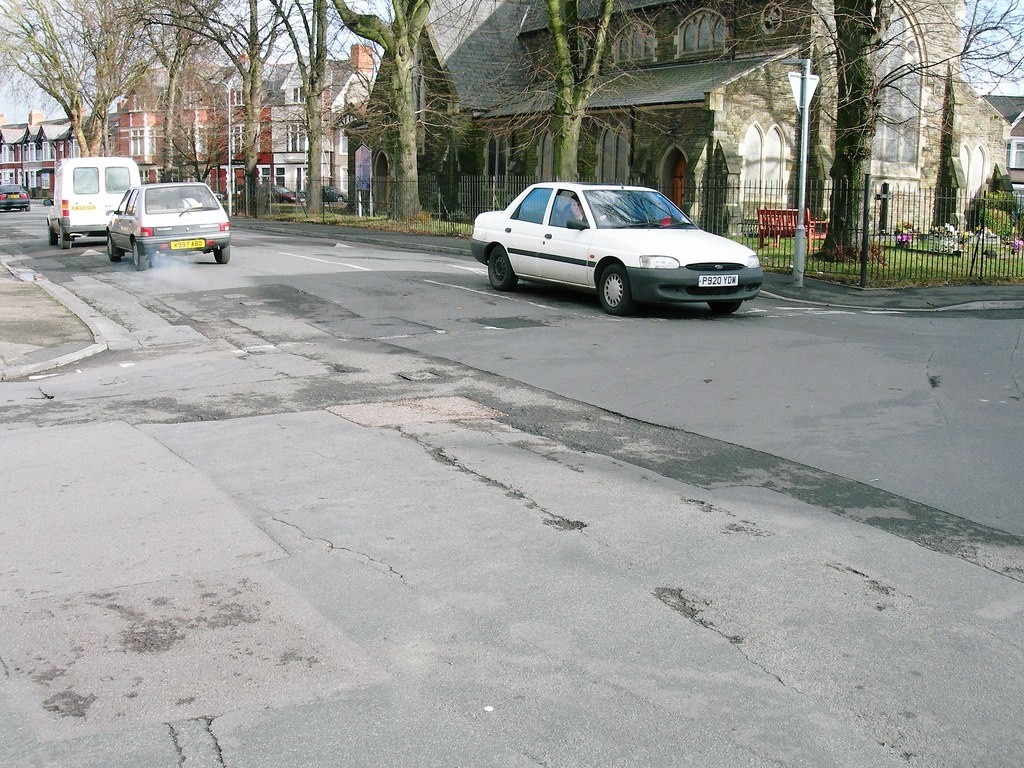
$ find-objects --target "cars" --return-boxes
[213,184,348,204]
[471,181,764,315]
[0,184,30,211]
[105,182,232,272]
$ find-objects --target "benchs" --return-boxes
[757,205,829,252]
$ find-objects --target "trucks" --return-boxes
[42,156,142,250]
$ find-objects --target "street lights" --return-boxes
[212,75,239,216]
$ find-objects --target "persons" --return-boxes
[570,194,606,222]
